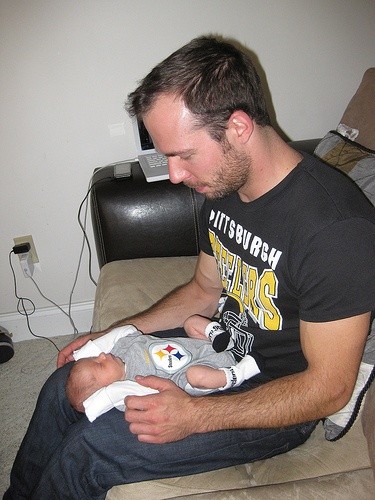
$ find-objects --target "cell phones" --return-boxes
[114,162,132,178]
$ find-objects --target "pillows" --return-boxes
[360,379,375,480]
[320,314,375,442]
[335,68,374,151]
[312,129,375,207]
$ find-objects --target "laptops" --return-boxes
[132,116,171,183]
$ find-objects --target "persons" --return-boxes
[3,37,375,499]
[64,313,268,423]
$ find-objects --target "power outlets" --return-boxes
[13,234,39,265]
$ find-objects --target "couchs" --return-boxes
[90,136,375,500]
